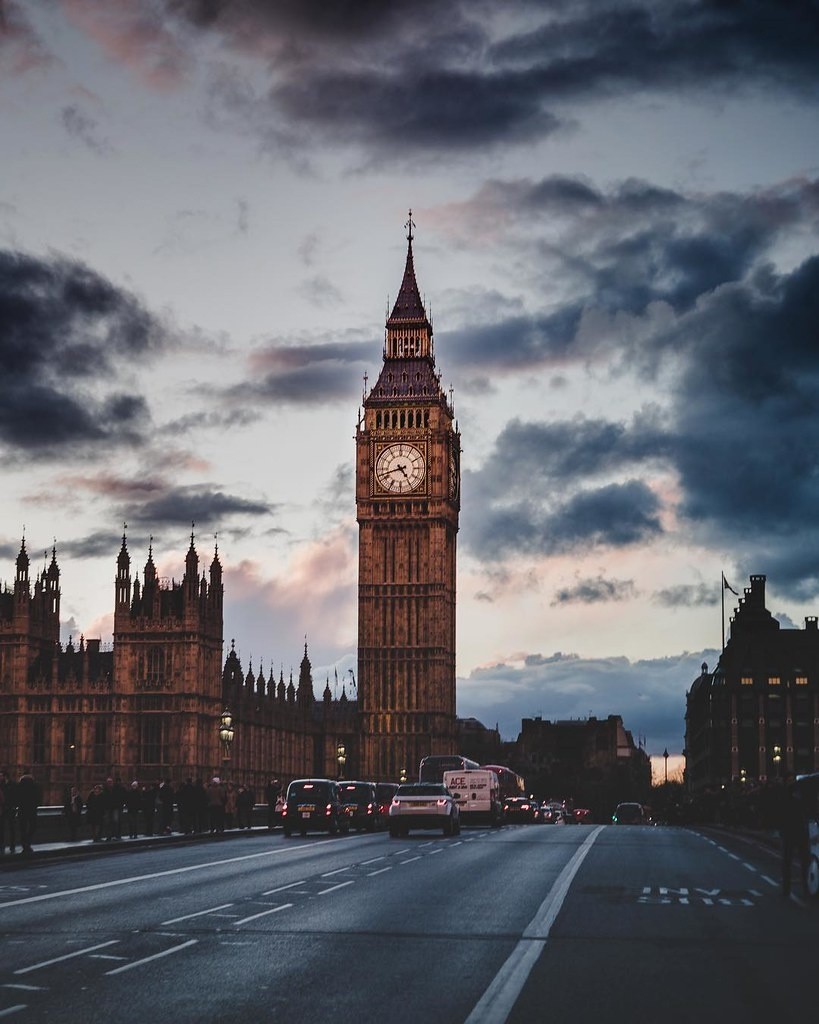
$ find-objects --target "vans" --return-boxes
[443,771,505,823]
[283,778,350,837]
[367,781,399,828]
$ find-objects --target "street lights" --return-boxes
[336,741,349,780]
[217,706,237,833]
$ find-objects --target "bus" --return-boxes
[418,755,482,786]
[472,764,526,802]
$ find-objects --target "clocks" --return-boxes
[374,442,427,495]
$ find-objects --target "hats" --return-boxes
[160,782,168,789]
[131,780,139,788]
[212,777,219,783]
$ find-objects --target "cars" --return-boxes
[613,801,645,827]
[338,782,378,832]
[388,782,462,836]
[501,795,593,826]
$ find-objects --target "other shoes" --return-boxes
[248,826,251,829]
[134,834,137,839]
[106,838,111,841]
[240,826,244,829]
[0,847,4,854]
[158,833,164,836]
[94,839,104,842]
[130,835,132,838]
[24,848,33,852]
[10,848,15,853]
[113,838,121,841]
[144,833,153,836]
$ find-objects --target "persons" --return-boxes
[0,774,285,856]
[774,772,811,898]
